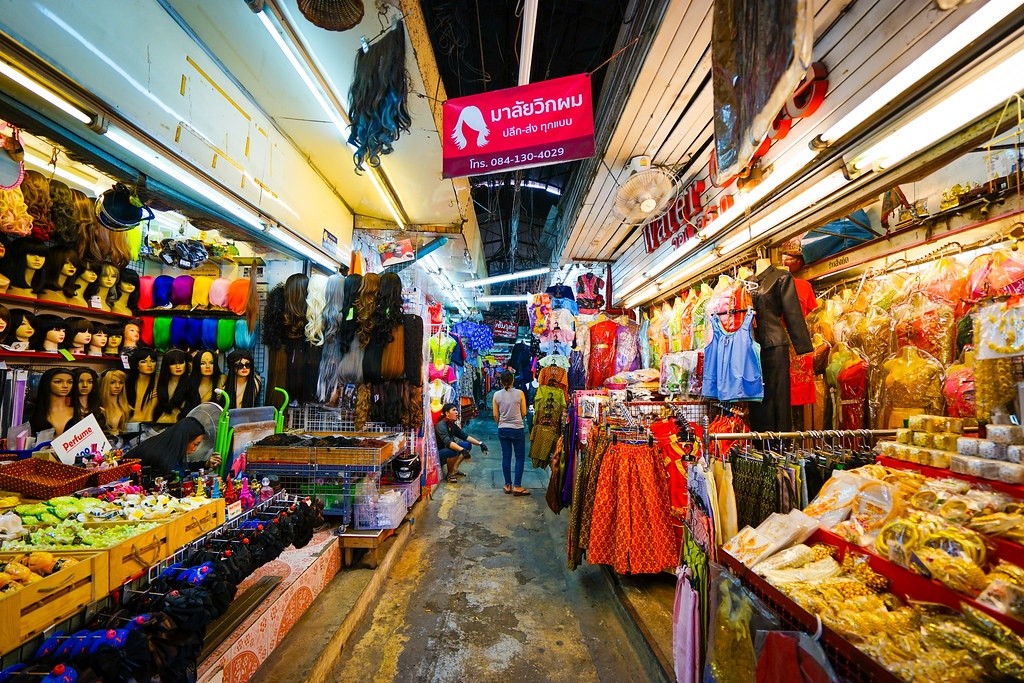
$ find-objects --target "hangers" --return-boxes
[593,393,879,473]
[535,267,640,430]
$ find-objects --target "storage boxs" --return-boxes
[0,453,227,658]
[247,431,421,530]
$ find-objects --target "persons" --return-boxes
[0,230,262,488]
[492,370,531,496]
[434,404,488,483]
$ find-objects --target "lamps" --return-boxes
[460,1,1024,310]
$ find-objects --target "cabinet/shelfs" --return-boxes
[714,454,1023,683]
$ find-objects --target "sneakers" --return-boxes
[446,474,457,483]
[454,470,466,478]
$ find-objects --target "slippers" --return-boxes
[502,485,512,494]
[513,488,530,496]
